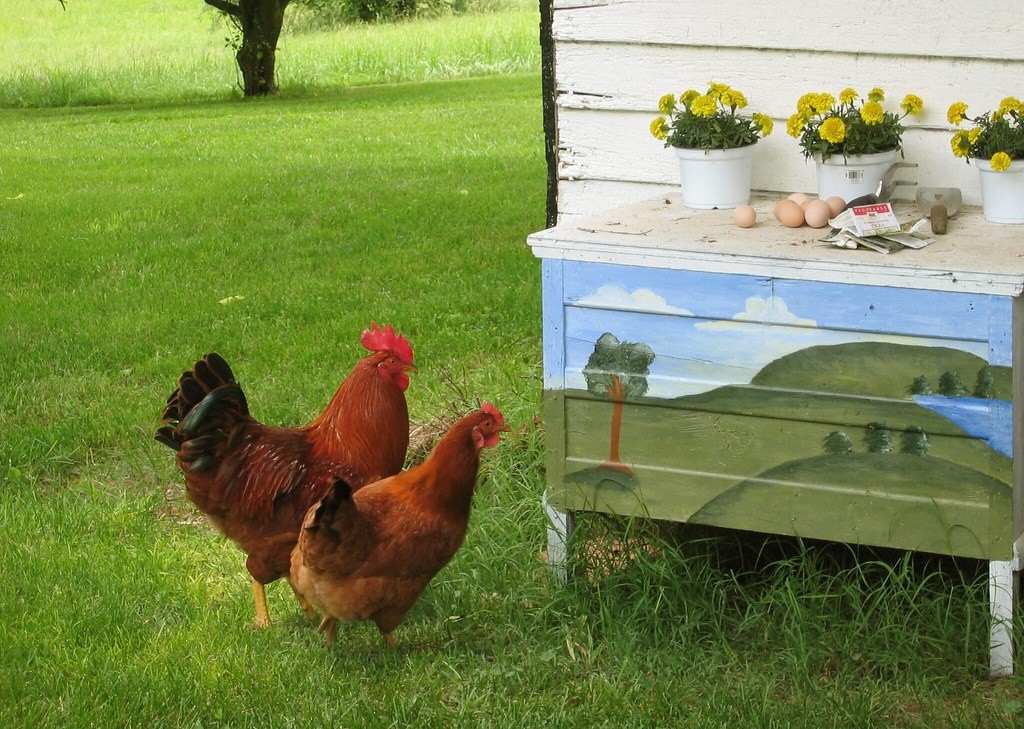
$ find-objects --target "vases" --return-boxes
[974,157,1024,225]
[672,141,759,210]
[812,149,898,204]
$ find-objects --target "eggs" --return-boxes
[733,205,756,228]
[773,192,846,228]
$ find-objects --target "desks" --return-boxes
[526,193,1024,677]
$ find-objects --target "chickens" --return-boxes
[153,323,513,648]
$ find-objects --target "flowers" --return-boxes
[788,86,924,165]
[650,80,774,155]
[947,96,1024,172]
